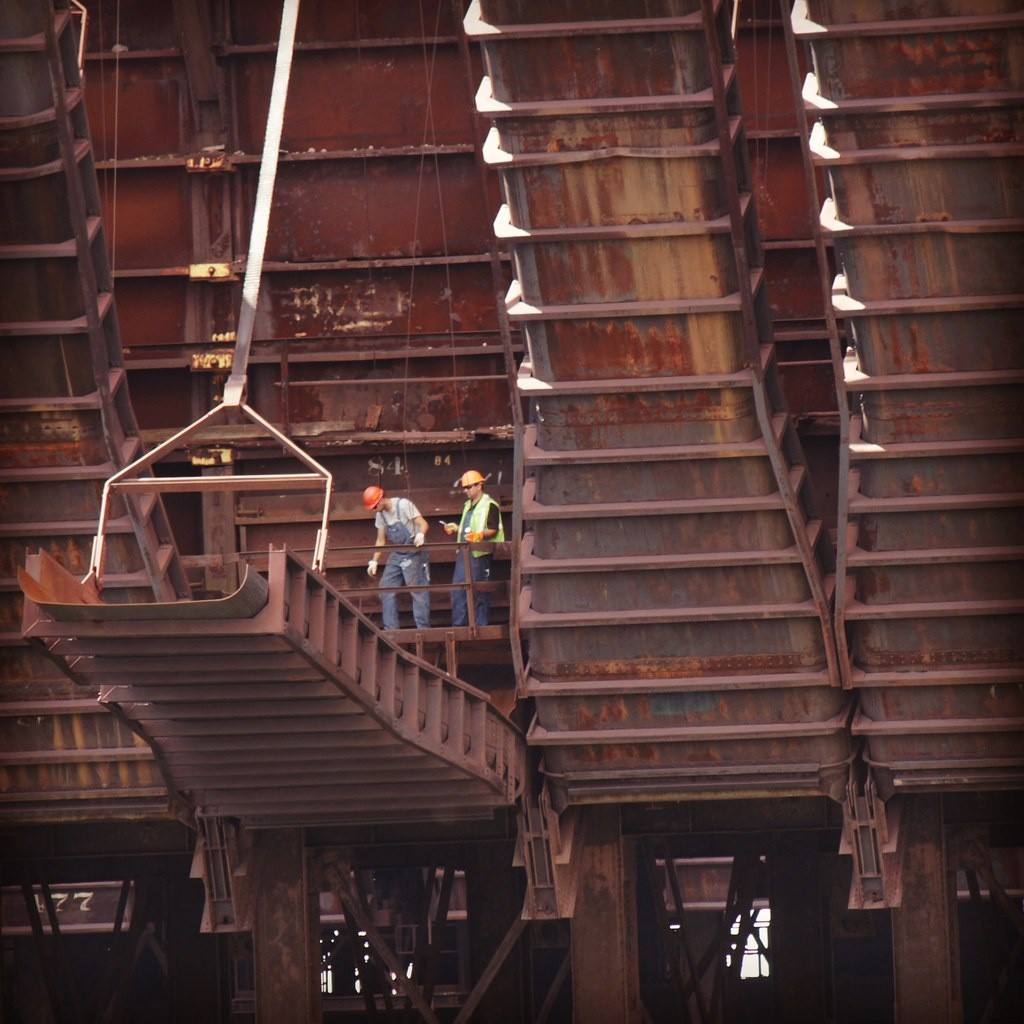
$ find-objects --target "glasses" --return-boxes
[373,505,377,509]
[464,483,478,489]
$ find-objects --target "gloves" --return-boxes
[464,532,482,543]
[410,532,425,547]
[444,523,458,535]
[367,561,378,579]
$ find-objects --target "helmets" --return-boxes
[462,470,485,486]
[363,486,384,510]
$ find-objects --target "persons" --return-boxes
[444,470,504,627]
[362,486,431,629]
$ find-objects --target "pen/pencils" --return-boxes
[439,521,447,526]
